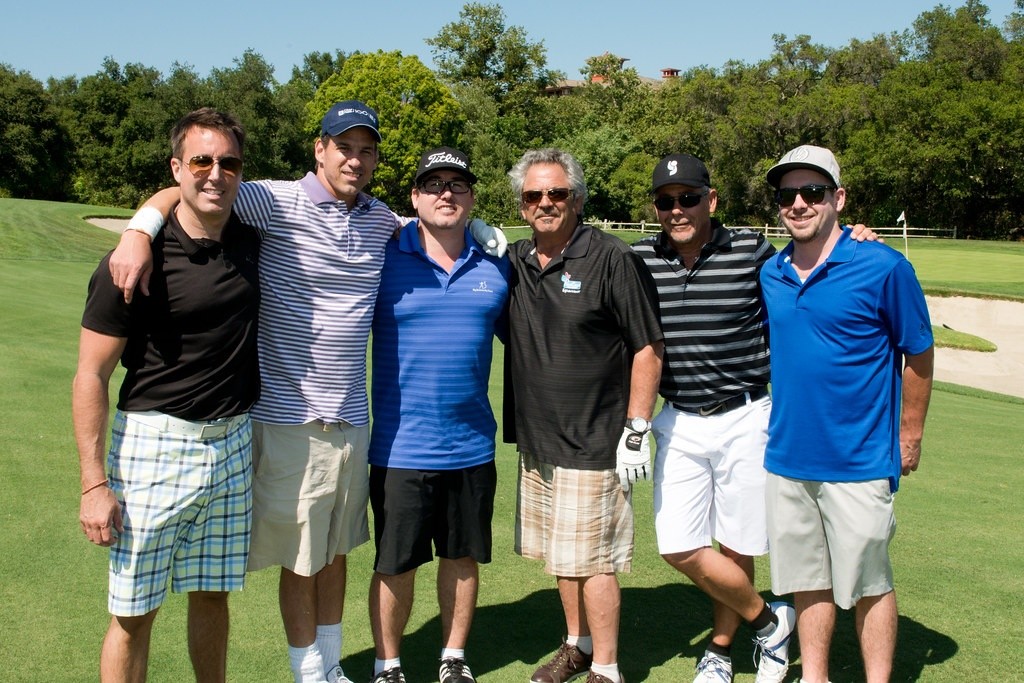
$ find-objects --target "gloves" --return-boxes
[615,427,651,492]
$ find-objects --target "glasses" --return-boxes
[521,188,575,203]
[652,191,709,211]
[773,184,837,207]
[177,155,243,179]
[416,178,474,194]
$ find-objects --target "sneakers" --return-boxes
[755,601,796,683]
[586,669,626,683]
[530,643,593,683]
[369,668,407,683]
[439,659,475,683]
[692,650,733,683]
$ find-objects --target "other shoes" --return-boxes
[326,665,356,683]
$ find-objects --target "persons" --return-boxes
[488,148,665,683]
[109,98,509,683]
[367,146,514,683]
[623,152,883,683]
[71,107,260,683]
[759,143,935,683]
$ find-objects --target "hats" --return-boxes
[321,101,382,143]
[649,154,711,197]
[416,146,478,183]
[766,144,841,190]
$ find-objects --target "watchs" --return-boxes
[624,417,652,433]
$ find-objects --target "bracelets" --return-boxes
[121,207,164,243]
[81,478,110,496]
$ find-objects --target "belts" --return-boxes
[665,385,769,417]
[121,409,246,439]
